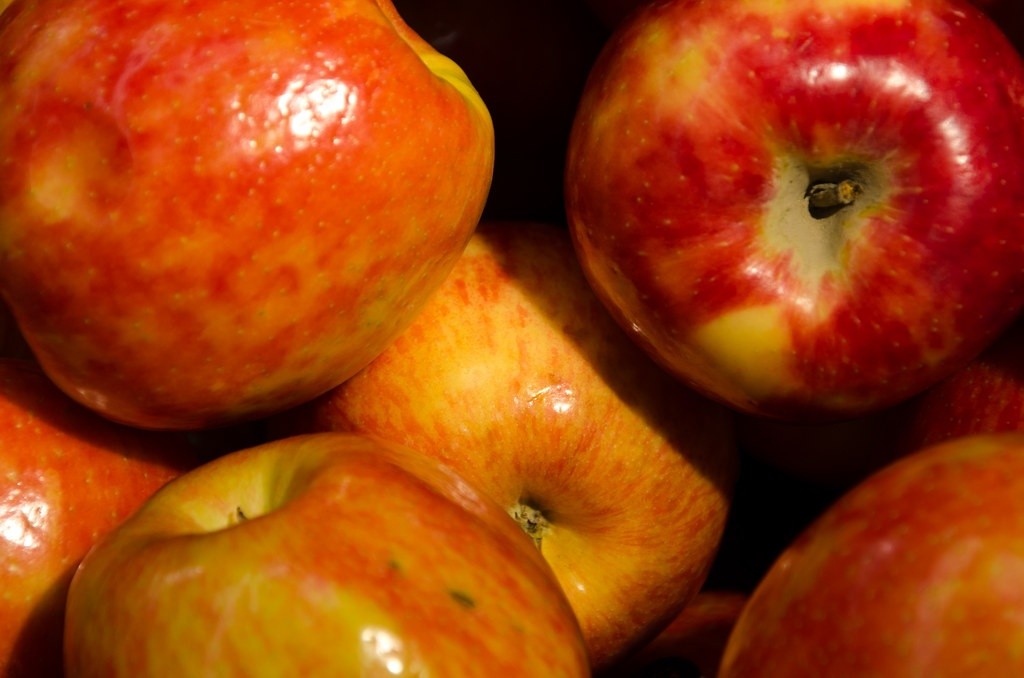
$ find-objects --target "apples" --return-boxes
[0,0,1024,678]
